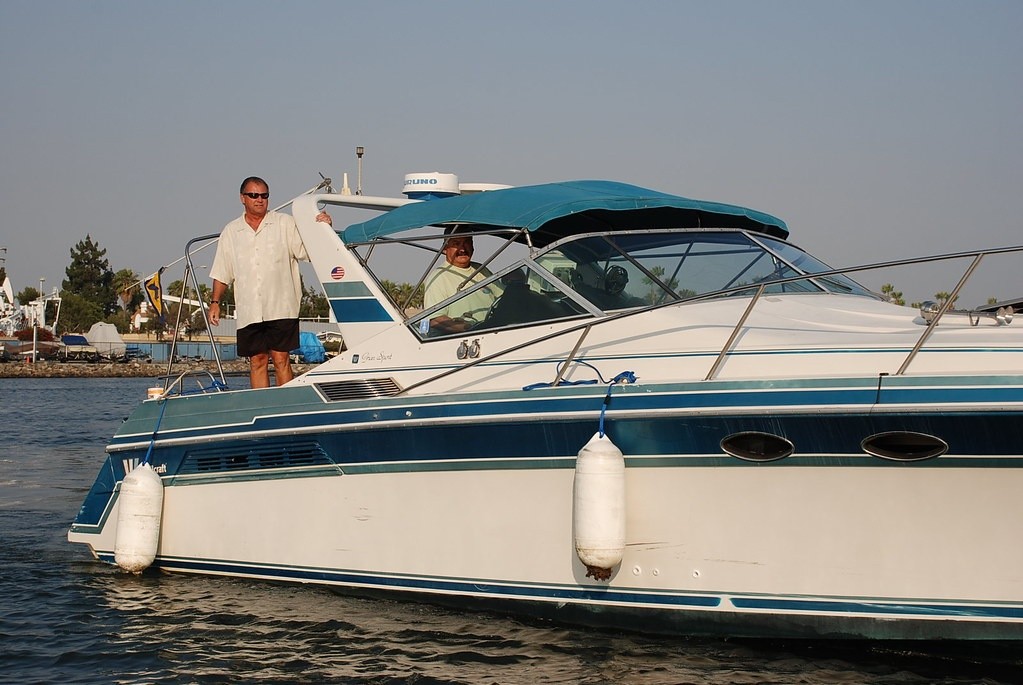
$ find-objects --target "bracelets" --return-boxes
[211,301,218,304]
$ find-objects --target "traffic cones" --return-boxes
[25,356,31,363]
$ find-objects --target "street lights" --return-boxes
[189,266,207,316]
[32,322,38,364]
[39,278,44,330]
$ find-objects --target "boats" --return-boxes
[59,334,96,362]
[18,333,65,358]
[82,321,126,357]
[67,172,1023,652]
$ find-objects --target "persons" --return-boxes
[208,176,332,389]
[423,226,506,334]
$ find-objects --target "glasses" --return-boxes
[243,192,269,199]
